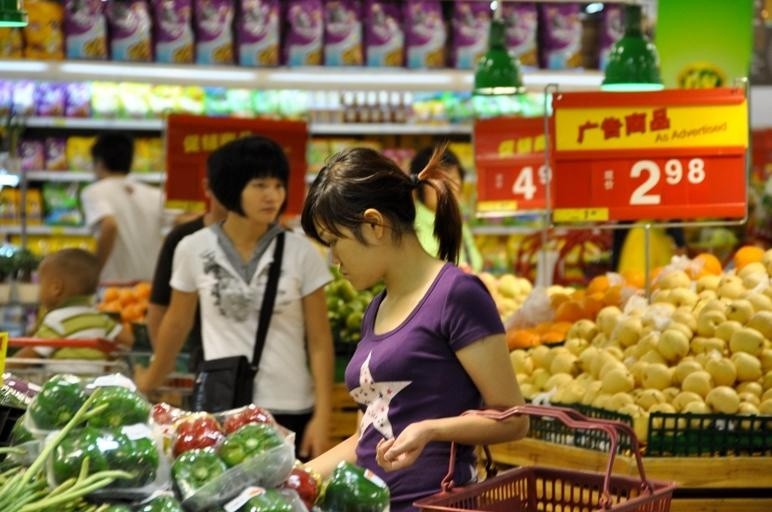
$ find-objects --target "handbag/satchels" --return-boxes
[191,356,255,412]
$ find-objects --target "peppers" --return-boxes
[324,265,386,340]
[14,374,392,511]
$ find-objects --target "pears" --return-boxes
[509,263,772,460]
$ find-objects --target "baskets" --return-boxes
[412,404,676,512]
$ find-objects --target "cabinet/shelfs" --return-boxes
[2,60,605,240]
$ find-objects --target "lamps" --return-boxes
[470,0,524,96]
[597,1,665,94]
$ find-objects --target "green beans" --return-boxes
[0,386,134,509]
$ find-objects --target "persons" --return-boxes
[78,130,168,283]
[301,146,531,512]
[146,176,228,373]
[132,133,337,465]
[3,248,135,368]
[410,144,484,273]
